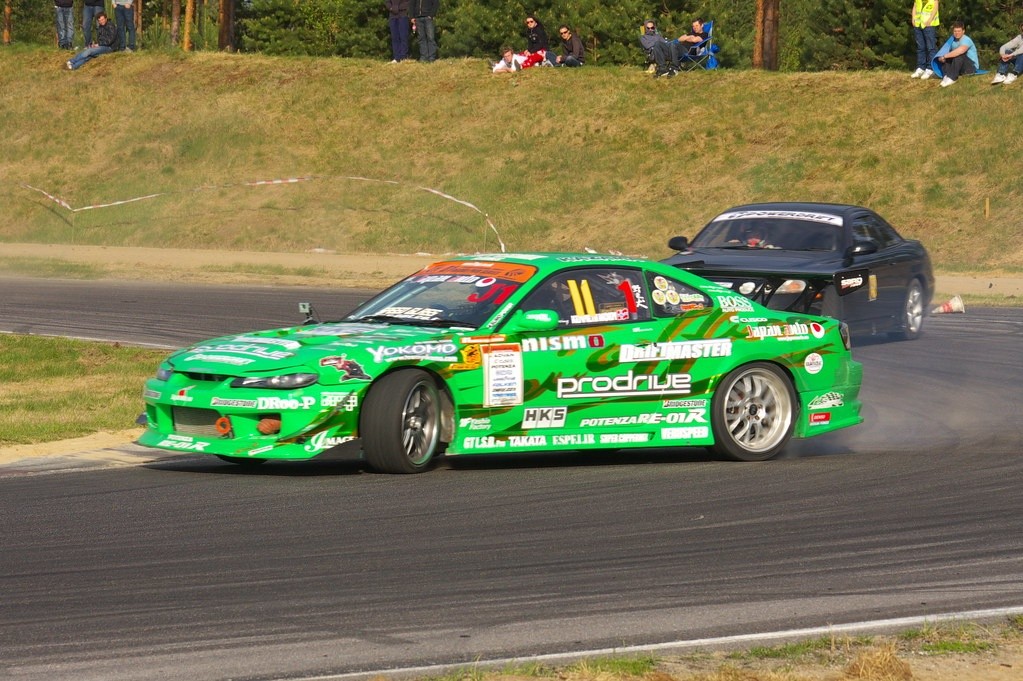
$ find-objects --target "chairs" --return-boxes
[678,19,716,73]
[637,24,673,72]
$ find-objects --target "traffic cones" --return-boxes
[931,294,966,315]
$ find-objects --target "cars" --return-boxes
[656,202,935,342]
[131,253,863,476]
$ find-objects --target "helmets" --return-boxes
[739,218,771,246]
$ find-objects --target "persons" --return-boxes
[386,0,437,64]
[112,0,135,54]
[939,22,979,87]
[64,12,119,70]
[910,0,939,80]
[493,15,585,73]
[991,23,1023,85]
[640,17,709,78]
[54,0,73,50]
[83,0,105,50]
[729,219,780,248]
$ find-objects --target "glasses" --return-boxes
[646,26,655,29]
[526,20,535,24]
[560,29,568,35]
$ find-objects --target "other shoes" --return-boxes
[487,59,494,69]
[514,59,521,71]
[653,69,669,78]
[939,75,957,87]
[667,68,679,78]
[921,69,934,79]
[911,68,924,78]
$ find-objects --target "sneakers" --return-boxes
[1003,73,1018,84]
[991,74,1006,84]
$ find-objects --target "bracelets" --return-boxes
[941,55,946,59]
